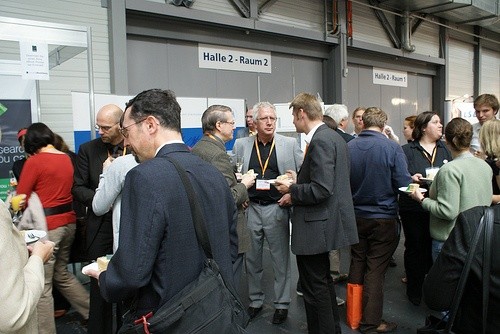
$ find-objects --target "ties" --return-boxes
[108,145,118,159]
[304,143,309,158]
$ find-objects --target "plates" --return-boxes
[82,262,99,277]
[265,179,293,186]
[236,173,258,181]
[24,229,47,243]
[419,178,433,182]
[398,187,427,192]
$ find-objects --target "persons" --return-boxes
[238,107,259,139]
[231,102,304,324]
[396,93,500,334]
[0,199,55,334]
[344,107,412,334]
[71,104,139,334]
[293,104,400,306]
[9,122,89,334]
[190,105,259,319]
[82,88,240,334]
[273,91,361,334]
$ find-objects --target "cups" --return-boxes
[426,168,439,180]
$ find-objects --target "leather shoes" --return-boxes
[273,309,287,323]
[248,307,263,319]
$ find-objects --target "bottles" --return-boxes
[8,170,18,192]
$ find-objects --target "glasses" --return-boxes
[215,120,235,128]
[257,117,277,122]
[118,118,159,138]
[94,121,120,132]
[245,116,252,119]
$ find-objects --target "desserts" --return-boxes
[409,184,419,191]
[97,256,110,272]
[276,175,289,184]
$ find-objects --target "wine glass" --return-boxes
[12,193,24,223]
[233,156,244,173]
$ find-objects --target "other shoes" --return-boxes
[401,278,406,283]
[389,257,397,267]
[54,309,69,318]
[410,298,421,306]
[367,320,397,334]
[297,291,303,296]
[334,273,348,284]
[336,297,344,305]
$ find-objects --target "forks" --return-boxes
[26,232,45,244]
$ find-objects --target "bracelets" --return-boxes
[419,197,425,205]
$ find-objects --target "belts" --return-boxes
[249,198,279,205]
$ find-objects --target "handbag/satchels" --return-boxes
[119,259,248,334]
[347,283,363,330]
[417,314,456,334]
